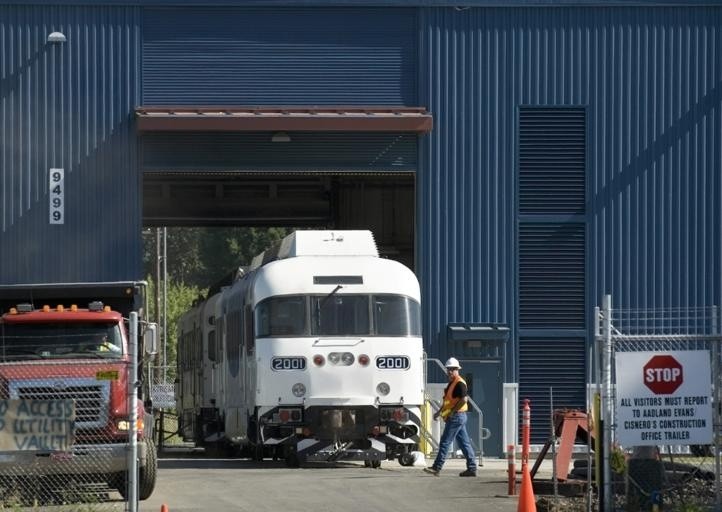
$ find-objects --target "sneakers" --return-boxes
[460,469,475,476]
[423,467,441,477]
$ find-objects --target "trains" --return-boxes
[172,228,426,470]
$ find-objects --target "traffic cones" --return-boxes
[516,462,538,511]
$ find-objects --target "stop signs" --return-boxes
[643,354,683,395]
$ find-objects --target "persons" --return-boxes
[422,357,477,477]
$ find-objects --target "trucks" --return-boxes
[0,276,161,500]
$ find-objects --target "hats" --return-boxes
[445,357,461,369]
